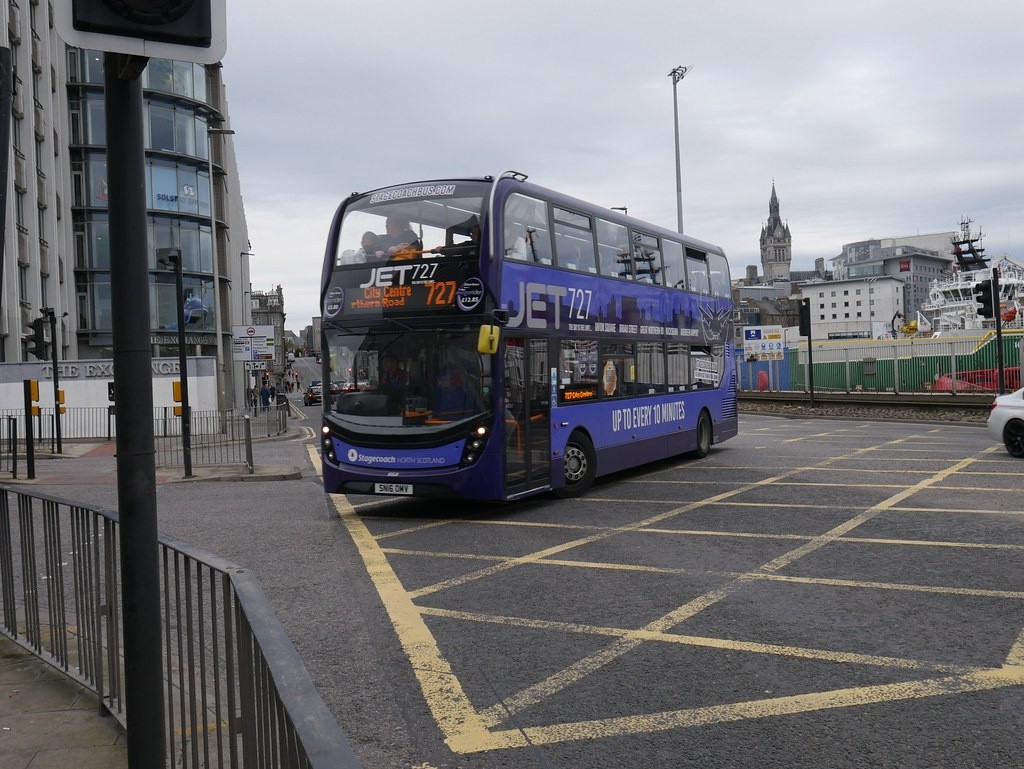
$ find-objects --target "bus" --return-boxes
[312,169,738,505]
[315,352,321,363]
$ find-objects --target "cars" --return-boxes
[303,386,323,406]
[307,380,321,389]
[987,387,1024,458]
[330,379,370,393]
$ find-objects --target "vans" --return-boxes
[285,352,296,362]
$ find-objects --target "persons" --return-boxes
[362,214,421,261]
[431,362,480,419]
[381,350,413,385]
[504,215,527,261]
[247,381,276,417]
[285,369,303,392]
[436,224,481,259]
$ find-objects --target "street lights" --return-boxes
[206,125,235,430]
[667,64,688,234]
[157,248,198,480]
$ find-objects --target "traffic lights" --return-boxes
[23,317,44,359]
[975,280,994,319]
